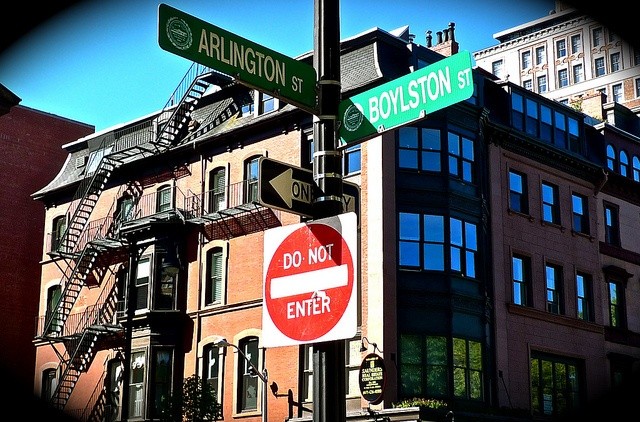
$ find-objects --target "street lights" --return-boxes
[213,337,268,422]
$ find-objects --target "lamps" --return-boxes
[359,337,377,352]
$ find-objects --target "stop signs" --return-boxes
[260,211,358,348]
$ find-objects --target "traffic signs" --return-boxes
[257,156,361,231]
[336,48,475,151]
[157,2,320,116]
